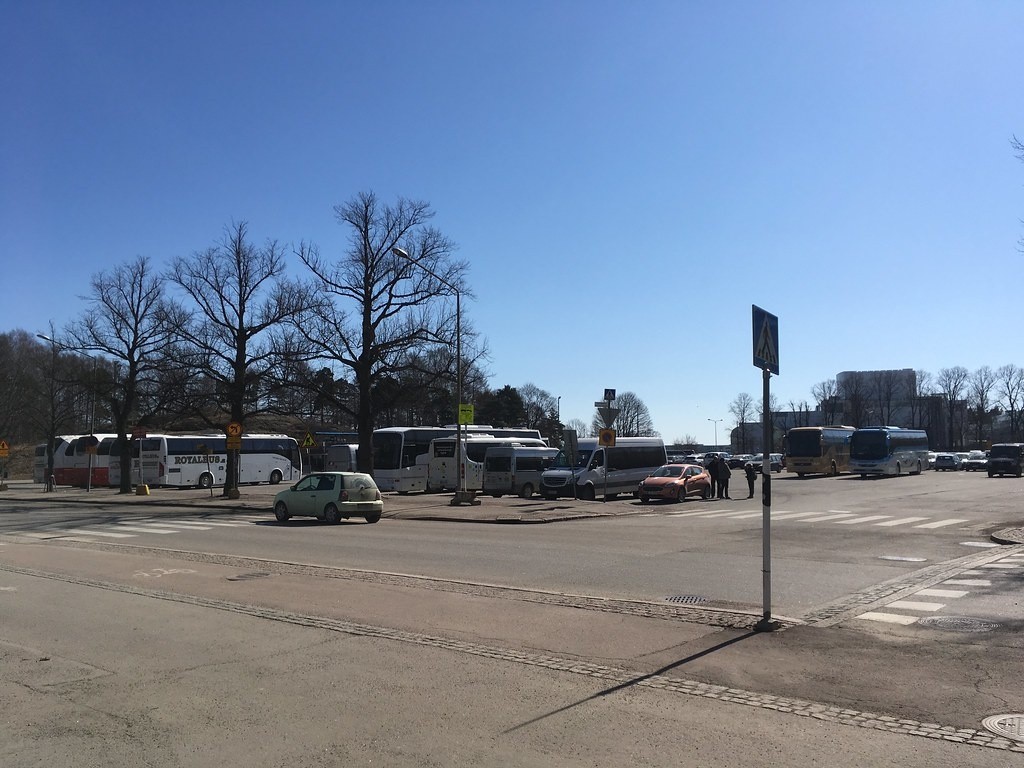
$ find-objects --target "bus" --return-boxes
[429,434,552,495]
[139,435,302,490]
[780,424,857,479]
[842,426,930,479]
[666,450,696,464]
[32,433,165,489]
[370,424,544,496]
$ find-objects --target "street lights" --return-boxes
[391,246,462,494]
[558,396,562,438]
[708,418,723,445]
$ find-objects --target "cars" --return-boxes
[927,443,1024,478]
[273,471,383,525]
[683,451,755,470]
[638,464,711,503]
[745,455,784,475]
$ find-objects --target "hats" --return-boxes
[720,458,725,461]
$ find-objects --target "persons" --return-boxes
[708,456,720,498]
[717,458,731,499]
[745,463,755,498]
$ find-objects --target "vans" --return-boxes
[482,445,566,499]
[325,444,361,473]
[536,437,668,502]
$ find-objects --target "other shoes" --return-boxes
[721,496,724,498]
[726,497,731,499]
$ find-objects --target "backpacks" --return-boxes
[751,468,757,480]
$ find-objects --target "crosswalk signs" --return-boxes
[751,304,779,376]
[604,389,616,401]
